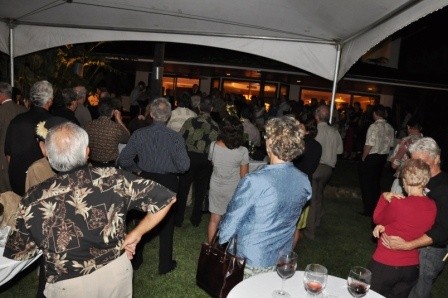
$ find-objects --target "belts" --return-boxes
[89,159,116,167]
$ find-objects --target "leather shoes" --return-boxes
[168,260,176,271]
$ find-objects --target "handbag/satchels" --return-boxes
[195,227,245,298]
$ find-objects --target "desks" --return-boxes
[226,271,386,298]
[0,225,45,298]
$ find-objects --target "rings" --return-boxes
[386,244,389,246]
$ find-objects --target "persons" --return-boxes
[362,157,437,298]
[118,97,191,277]
[302,105,344,241]
[219,114,312,283]
[1,77,447,227]
[290,114,322,249]
[373,137,448,298]
[208,114,250,245]
[1,122,177,298]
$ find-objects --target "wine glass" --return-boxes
[303,264,328,298]
[248,140,257,161]
[273,250,298,298]
[346,266,372,298]
